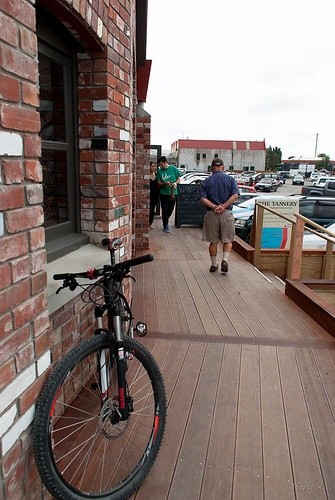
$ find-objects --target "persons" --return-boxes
[157,156,181,232]
[255,176,261,184]
[241,171,244,178]
[149,161,157,229]
[197,157,240,272]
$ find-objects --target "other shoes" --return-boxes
[210,264,218,272]
[150,225,154,229]
[164,225,170,232]
[221,260,228,272]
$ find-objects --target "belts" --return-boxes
[225,208,232,210]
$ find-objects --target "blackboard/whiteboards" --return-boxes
[175,183,207,228]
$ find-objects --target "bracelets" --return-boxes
[213,204,215,210]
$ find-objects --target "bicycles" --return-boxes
[30,237,169,500]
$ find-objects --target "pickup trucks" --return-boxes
[301,180,335,197]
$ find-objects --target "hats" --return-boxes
[212,157,224,166]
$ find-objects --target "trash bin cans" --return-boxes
[250,166,254,171]
[243,167,249,171]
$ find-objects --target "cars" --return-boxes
[292,174,304,185]
[237,185,257,194]
[177,168,290,187]
[313,177,335,187]
[255,178,278,192]
[230,193,335,250]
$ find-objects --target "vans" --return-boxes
[309,172,328,182]
[297,171,306,179]
